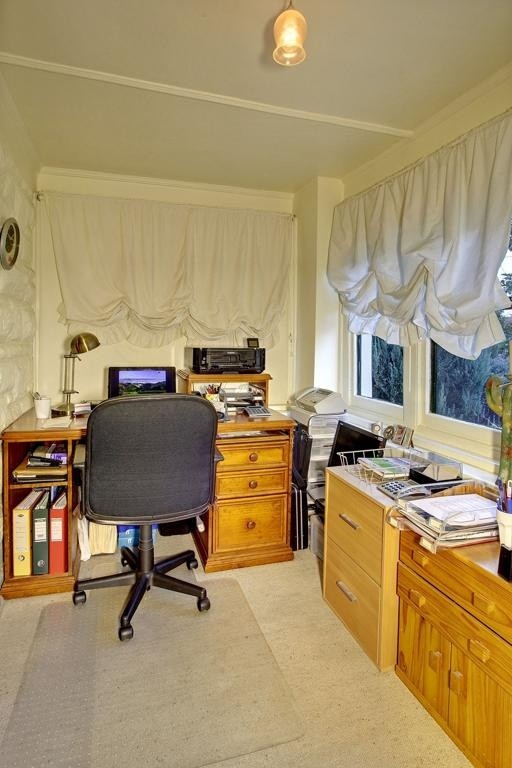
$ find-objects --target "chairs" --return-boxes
[62,394,223,639]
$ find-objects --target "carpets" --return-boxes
[0,578,307,768]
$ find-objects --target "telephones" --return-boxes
[294,386,344,415]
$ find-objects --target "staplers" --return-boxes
[27,455,62,467]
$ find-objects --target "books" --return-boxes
[12,444,67,484]
[355,446,501,544]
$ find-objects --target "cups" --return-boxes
[34,397,50,419]
[206,394,220,402]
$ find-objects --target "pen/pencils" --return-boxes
[496,478,512,514]
[29,390,41,400]
[205,382,222,394]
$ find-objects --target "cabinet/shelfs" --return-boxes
[321,465,431,672]
[394,527,512,768]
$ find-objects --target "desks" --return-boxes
[0,369,296,600]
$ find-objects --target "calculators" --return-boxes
[244,406,271,418]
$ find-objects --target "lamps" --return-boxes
[52,333,101,417]
[272,0,308,66]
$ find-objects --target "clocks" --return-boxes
[0,217,20,270]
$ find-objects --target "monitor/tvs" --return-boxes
[108,365,176,397]
[327,421,387,480]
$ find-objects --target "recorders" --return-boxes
[192,336,266,375]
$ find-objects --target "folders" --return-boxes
[12,490,68,577]
